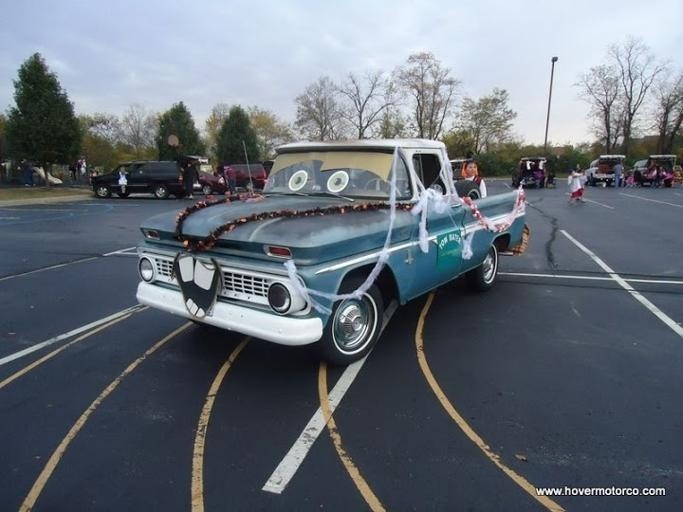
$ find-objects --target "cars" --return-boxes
[1,159,26,170]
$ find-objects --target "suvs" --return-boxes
[584,155,626,186]
[513,156,554,187]
[92,156,266,200]
[628,154,677,187]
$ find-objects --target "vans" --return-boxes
[197,163,267,195]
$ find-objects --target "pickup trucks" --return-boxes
[136,138,528,364]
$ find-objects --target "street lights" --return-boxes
[542,57,559,153]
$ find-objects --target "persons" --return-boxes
[69,155,97,187]
[531,160,674,205]
[23,158,35,186]
[461,160,487,198]
[225,162,237,195]
[181,162,195,199]
[117,167,129,198]
